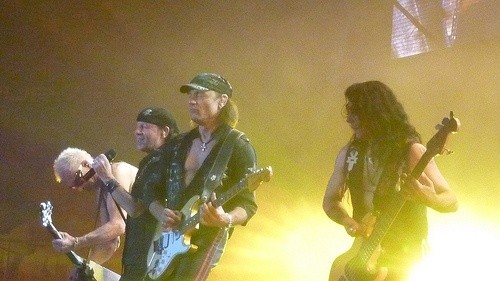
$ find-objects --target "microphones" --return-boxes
[79,148,118,183]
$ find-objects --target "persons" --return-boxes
[141,72,258,281]
[51,147,139,264]
[91,107,180,281]
[322,80,459,281]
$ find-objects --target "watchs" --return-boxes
[74,237,79,248]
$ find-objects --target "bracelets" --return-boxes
[105,178,122,193]
[220,213,232,232]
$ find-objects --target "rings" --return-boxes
[162,220,164,225]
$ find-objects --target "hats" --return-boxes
[137,107,179,133]
[180,73,232,99]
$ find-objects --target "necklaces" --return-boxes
[199,134,214,152]
[137,156,157,179]
[365,152,380,184]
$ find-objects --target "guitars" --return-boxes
[324,110,461,281]
[38,200,97,281]
[145,164,273,281]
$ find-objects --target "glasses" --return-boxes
[69,163,83,190]
